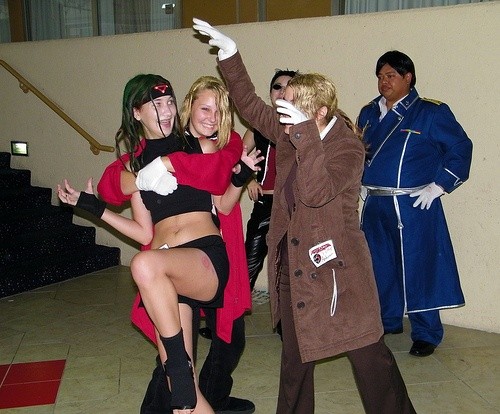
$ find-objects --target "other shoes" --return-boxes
[217,396,255,413]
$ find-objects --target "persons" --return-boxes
[356,51,474,357]
[58,17,419,414]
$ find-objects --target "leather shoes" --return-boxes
[384,328,401,334]
[408,340,435,356]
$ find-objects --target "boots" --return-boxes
[159,328,197,412]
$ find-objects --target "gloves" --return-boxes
[192,17,237,61]
[275,99,308,125]
[135,156,178,196]
[409,181,446,210]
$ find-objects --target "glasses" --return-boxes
[272,84,284,91]
